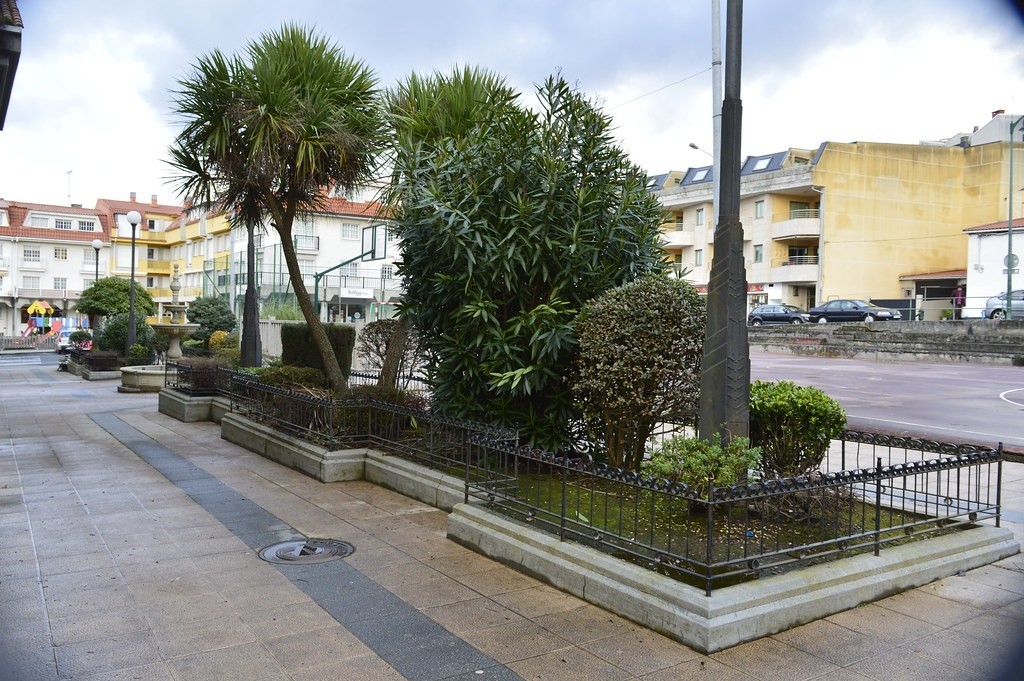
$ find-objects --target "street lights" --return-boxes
[91,239,102,284]
[125,210,141,356]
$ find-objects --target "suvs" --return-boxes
[748,305,810,326]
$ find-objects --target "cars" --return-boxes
[985,290,1024,319]
[808,299,902,323]
